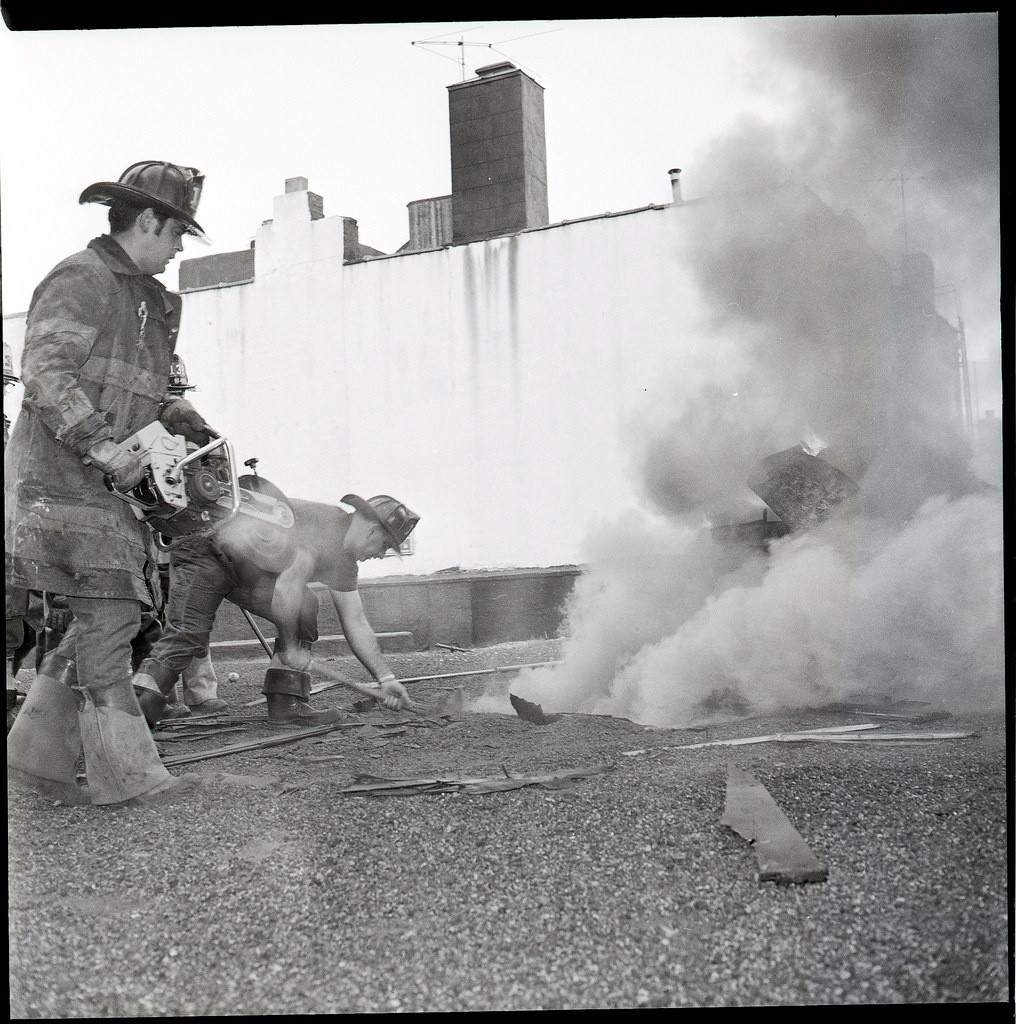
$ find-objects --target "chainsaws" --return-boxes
[103,419,300,574]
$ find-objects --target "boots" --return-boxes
[261,668,340,726]
[169,700,230,717]
[131,684,168,751]
[7,651,201,805]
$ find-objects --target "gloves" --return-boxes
[86,443,146,495]
[164,400,211,451]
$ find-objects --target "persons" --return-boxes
[132,494,419,757]
[4,342,227,719]
[4,161,210,808]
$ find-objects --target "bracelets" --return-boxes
[378,675,395,684]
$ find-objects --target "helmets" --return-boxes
[341,494,422,555]
[78,161,206,237]
